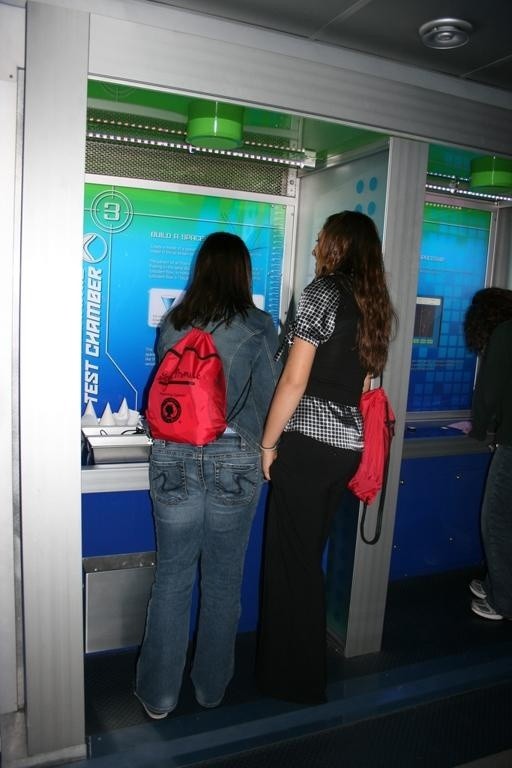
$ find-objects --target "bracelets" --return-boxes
[260,443,278,451]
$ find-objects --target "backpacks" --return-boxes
[145,306,256,446]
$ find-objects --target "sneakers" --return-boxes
[468,580,487,599]
[471,598,503,620]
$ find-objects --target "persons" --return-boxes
[465,288,511,620]
[134,232,284,721]
[259,211,402,702]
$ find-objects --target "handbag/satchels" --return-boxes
[347,387,396,505]
